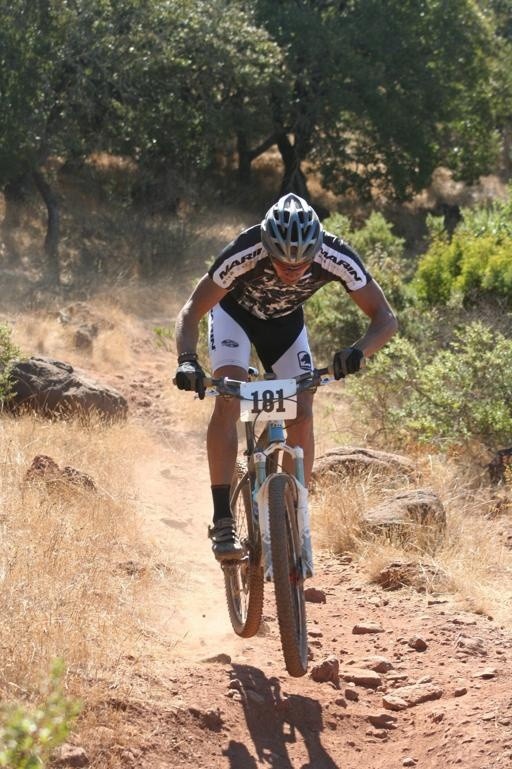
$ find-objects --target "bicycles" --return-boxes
[170,360,336,679]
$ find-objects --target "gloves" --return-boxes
[333,346,364,380]
[175,359,206,400]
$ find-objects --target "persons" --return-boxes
[175,193,397,561]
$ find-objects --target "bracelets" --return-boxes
[178,351,198,363]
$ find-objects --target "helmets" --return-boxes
[259,192,324,270]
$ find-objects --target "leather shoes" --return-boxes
[207,518,244,561]
